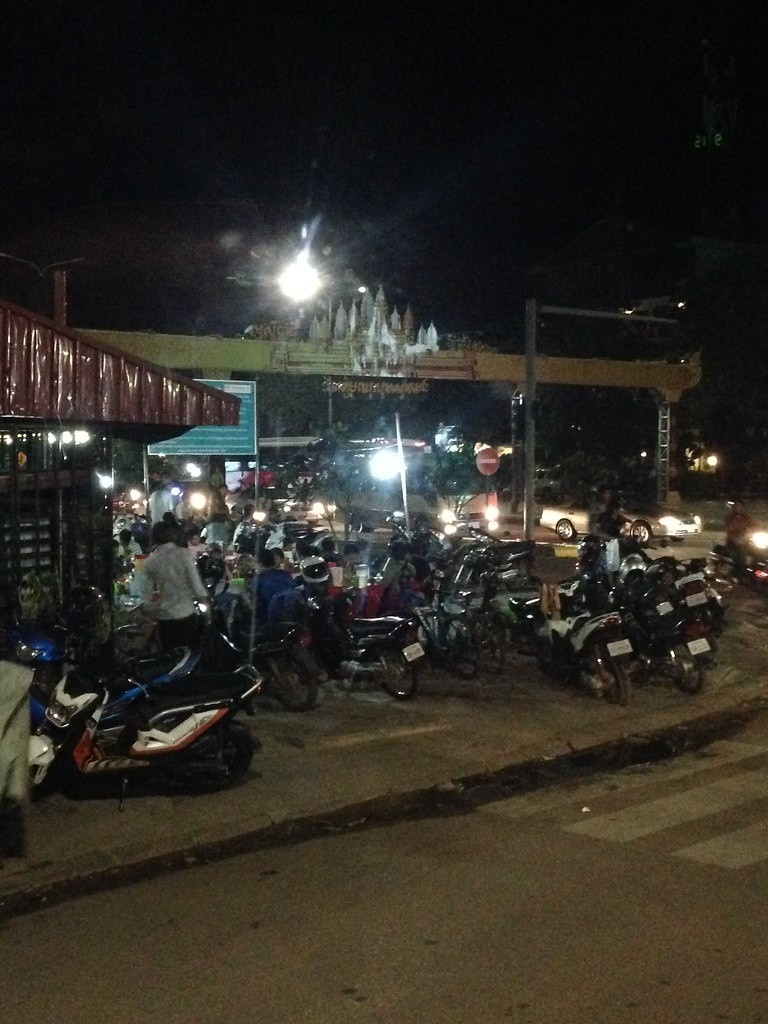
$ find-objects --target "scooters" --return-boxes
[0,512,768,813]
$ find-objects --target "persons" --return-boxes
[588,485,633,539]
[542,534,614,614]
[724,501,764,584]
[112,468,444,636]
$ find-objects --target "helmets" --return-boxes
[63,588,103,632]
[300,557,329,583]
[619,554,645,583]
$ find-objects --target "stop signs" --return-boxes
[477,448,500,475]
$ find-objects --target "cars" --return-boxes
[538,493,700,547]
